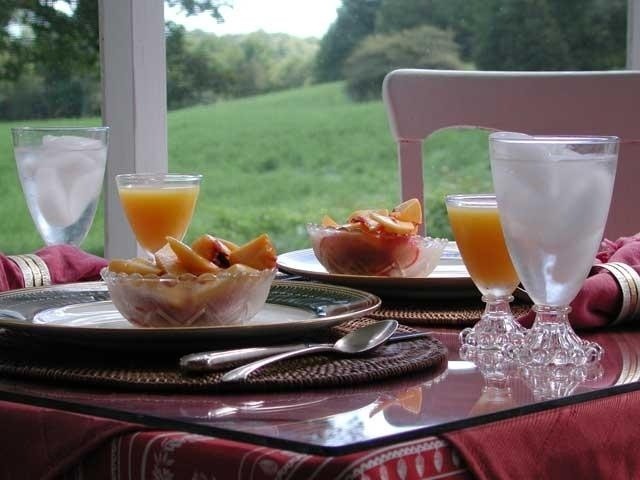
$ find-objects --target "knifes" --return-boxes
[178,330,434,376]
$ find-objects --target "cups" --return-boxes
[114,172,204,255]
[9,126,111,249]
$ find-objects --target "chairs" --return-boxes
[381,65,640,241]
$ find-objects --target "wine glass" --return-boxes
[488,133,622,365]
[448,192,531,351]
[456,344,524,424]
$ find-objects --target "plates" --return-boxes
[276,240,474,296]
[0,280,384,347]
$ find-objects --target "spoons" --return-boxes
[219,319,400,382]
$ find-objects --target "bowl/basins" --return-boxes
[99,265,278,327]
[304,221,449,277]
